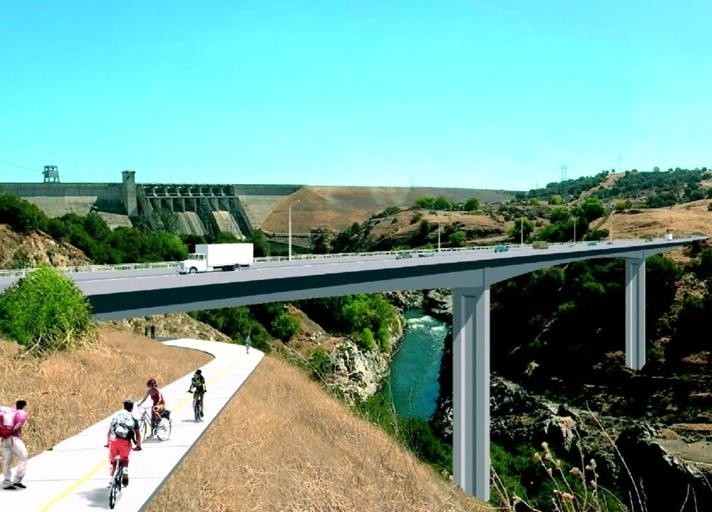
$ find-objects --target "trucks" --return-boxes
[176,242,256,274]
[533,241,549,249]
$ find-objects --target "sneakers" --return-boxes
[3,483,27,491]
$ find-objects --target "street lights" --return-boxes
[287,199,301,259]
[429,211,441,253]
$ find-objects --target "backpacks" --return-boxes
[112,409,136,440]
[0,406,24,438]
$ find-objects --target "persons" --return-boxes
[104,400,142,488]
[189,368,207,418]
[137,378,166,439]
[0,398,29,489]
[145,325,148,335]
[150,324,155,339]
[244,335,252,355]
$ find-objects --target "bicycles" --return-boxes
[132,403,171,445]
[188,389,206,423]
[104,444,142,510]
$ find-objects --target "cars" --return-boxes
[395,249,432,259]
[495,245,509,253]
